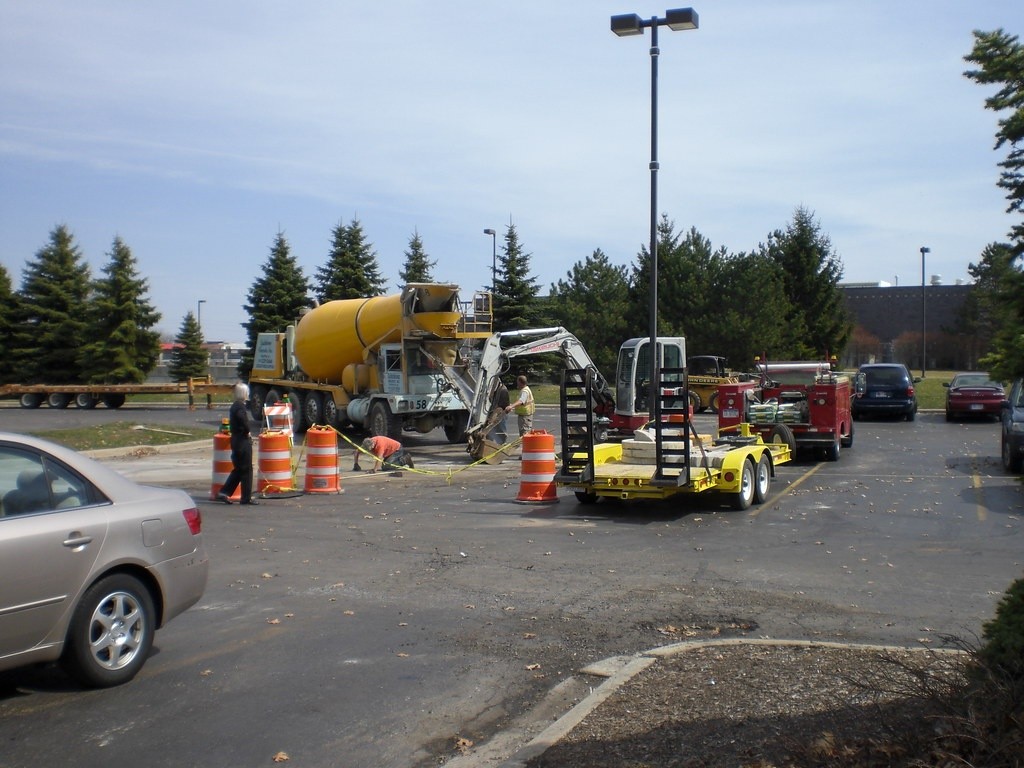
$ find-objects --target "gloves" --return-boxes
[353,463,362,471]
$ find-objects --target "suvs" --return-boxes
[849,361,923,422]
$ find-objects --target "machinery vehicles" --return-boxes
[682,352,738,414]
[245,278,498,442]
[462,326,698,477]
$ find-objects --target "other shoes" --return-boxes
[240,498,259,505]
[398,452,414,469]
[519,456,522,460]
[216,493,233,505]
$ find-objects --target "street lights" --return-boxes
[608,6,700,429]
[920,247,931,378]
[484,228,497,297]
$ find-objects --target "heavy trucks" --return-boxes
[552,350,868,514]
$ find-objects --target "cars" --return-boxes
[1002,374,1024,473]
[943,371,1007,423]
[1,429,210,689]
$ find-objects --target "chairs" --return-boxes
[959,380,968,385]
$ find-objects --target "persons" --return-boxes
[353,436,414,474]
[505,376,533,437]
[214,383,259,505]
[494,378,510,444]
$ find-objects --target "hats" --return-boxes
[361,437,375,451]
